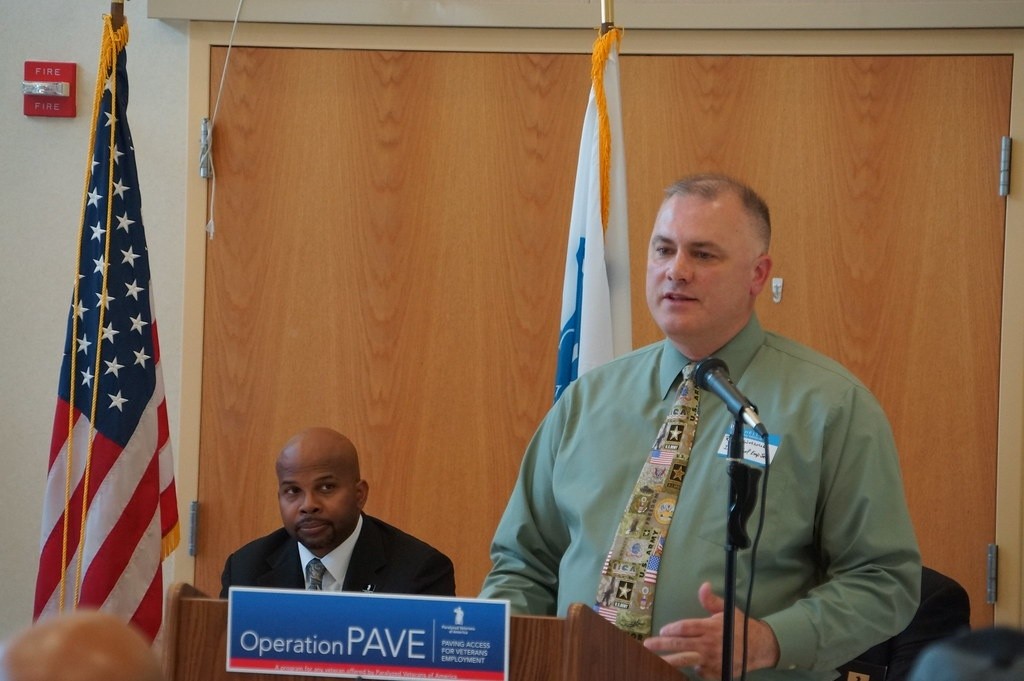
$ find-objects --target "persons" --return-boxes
[834,565,970,681]
[908,624,1024,681]
[219,428,456,599]
[0,610,164,681]
[478,171,923,681]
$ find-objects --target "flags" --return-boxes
[31,17,183,652]
[552,29,633,406]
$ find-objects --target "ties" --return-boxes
[306,558,326,591]
[591,362,701,647]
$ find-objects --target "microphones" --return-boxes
[692,357,769,438]
